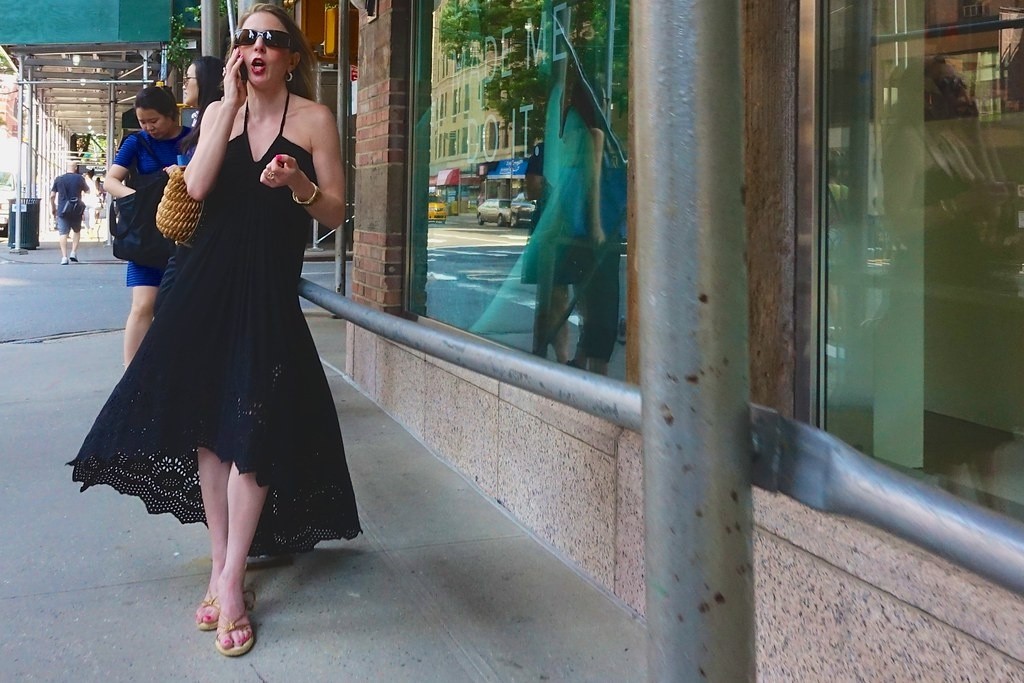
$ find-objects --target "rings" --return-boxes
[267,172,274,180]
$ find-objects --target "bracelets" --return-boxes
[292,182,320,205]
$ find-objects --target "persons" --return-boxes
[50,161,89,265]
[523,75,620,374]
[101,85,192,369]
[179,56,224,155]
[81,168,103,239]
[72,2,363,656]
[881,56,1010,284]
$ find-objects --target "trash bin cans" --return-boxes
[7,198,42,250]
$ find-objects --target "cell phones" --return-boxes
[234,45,248,84]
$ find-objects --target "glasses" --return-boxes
[183,75,198,84]
[232,27,293,50]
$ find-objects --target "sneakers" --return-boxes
[61,257,68,265]
[70,252,79,262]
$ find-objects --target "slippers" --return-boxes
[216,611,255,657]
[195,584,220,630]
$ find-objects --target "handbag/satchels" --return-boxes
[60,195,86,219]
[156,164,204,245]
[106,132,170,243]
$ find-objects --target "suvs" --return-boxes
[510,190,537,228]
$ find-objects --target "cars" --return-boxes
[428,193,449,224]
[476,196,512,227]
